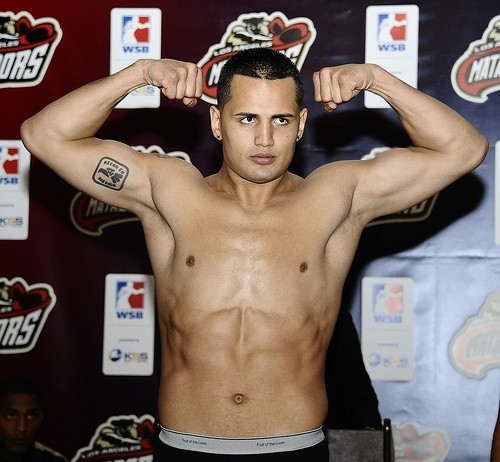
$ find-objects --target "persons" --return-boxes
[18,46,489,462]
